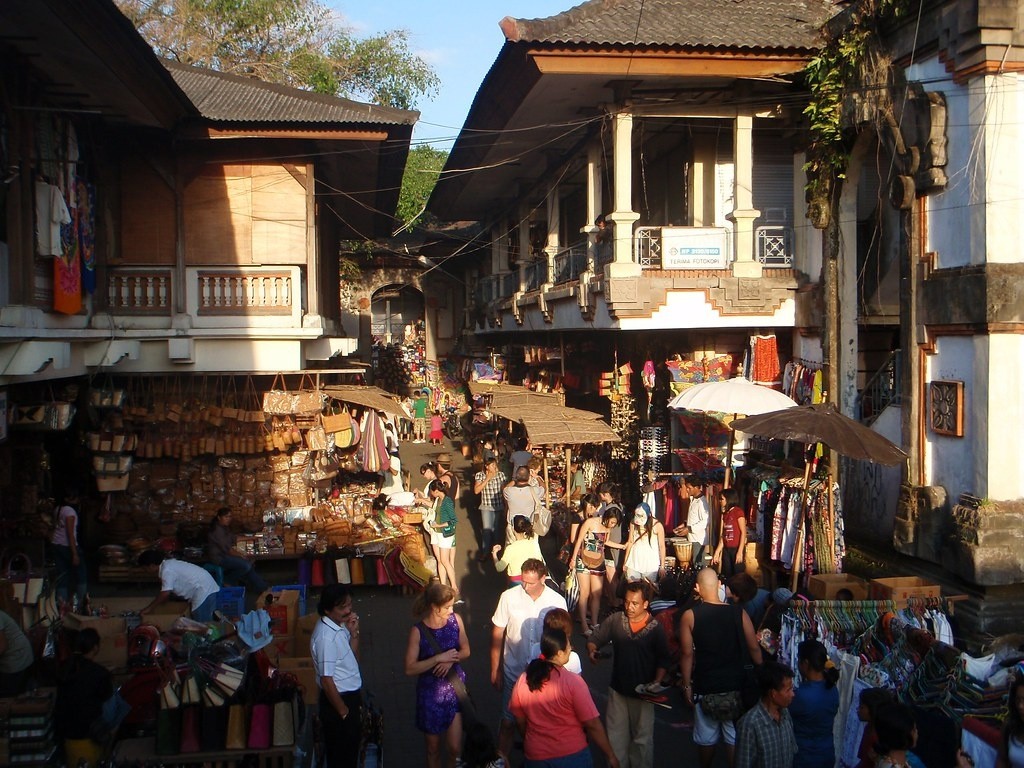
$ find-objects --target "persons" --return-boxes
[528,457,1024,768]
[474,457,509,563]
[491,515,546,589]
[492,629,619,768]
[490,559,574,768]
[398,390,462,447]
[503,467,547,551]
[375,445,462,597]
[309,583,368,768]
[405,584,470,768]
[468,422,529,482]
[0,484,301,768]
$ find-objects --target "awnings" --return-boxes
[524,417,622,445]
[484,407,604,423]
[314,385,410,420]
[467,381,566,406]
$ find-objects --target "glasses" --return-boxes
[421,471,426,475]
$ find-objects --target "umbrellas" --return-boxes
[665,374,799,575]
[727,402,911,599]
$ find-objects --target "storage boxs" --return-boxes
[279,657,320,704]
[141,596,191,631]
[272,584,307,617]
[67,611,127,669]
[256,589,300,637]
[808,572,870,600]
[869,576,941,601]
[295,612,361,662]
[218,585,247,618]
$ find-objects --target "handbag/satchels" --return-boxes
[700,691,744,720]
[456,672,488,709]
[564,568,580,613]
[403,500,422,523]
[119,650,294,755]
[85,371,352,493]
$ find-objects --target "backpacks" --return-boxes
[529,485,552,536]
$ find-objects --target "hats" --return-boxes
[435,453,452,464]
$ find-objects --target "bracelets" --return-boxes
[491,552,497,553]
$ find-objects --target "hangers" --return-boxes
[777,595,1024,723]
[796,356,824,371]
[745,460,835,493]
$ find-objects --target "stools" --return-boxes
[204,563,226,587]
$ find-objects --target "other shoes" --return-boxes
[454,595,465,604]
[412,439,427,444]
[581,624,599,637]
[480,553,488,561]
